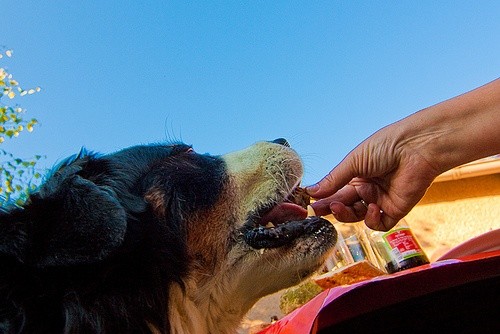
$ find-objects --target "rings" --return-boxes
[360,200,368,209]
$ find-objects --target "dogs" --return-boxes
[0,116,338,334]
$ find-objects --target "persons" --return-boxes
[305,78,500,232]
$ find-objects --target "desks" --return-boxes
[312,256,500,334]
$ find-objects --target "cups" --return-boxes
[322,224,381,273]
[364,218,429,272]
